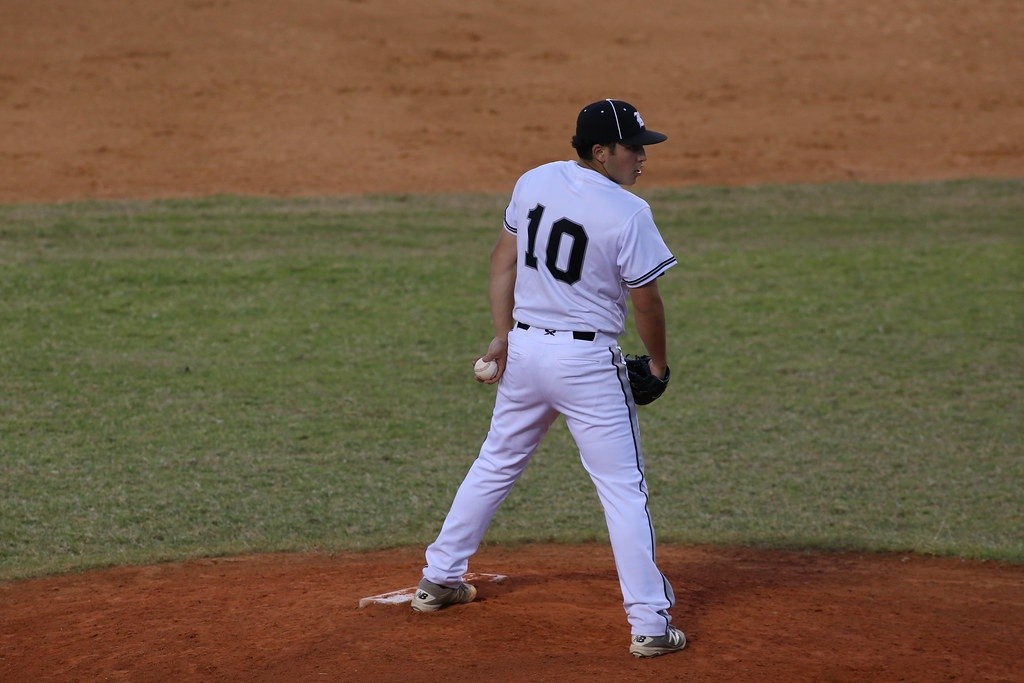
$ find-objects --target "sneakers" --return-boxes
[629,624,686,659]
[411,577,477,613]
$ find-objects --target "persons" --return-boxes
[413,100,692,658]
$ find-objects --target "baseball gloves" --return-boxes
[624,354,670,405]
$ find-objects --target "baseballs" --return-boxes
[474,358,498,380]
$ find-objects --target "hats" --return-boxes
[576,98,669,145]
